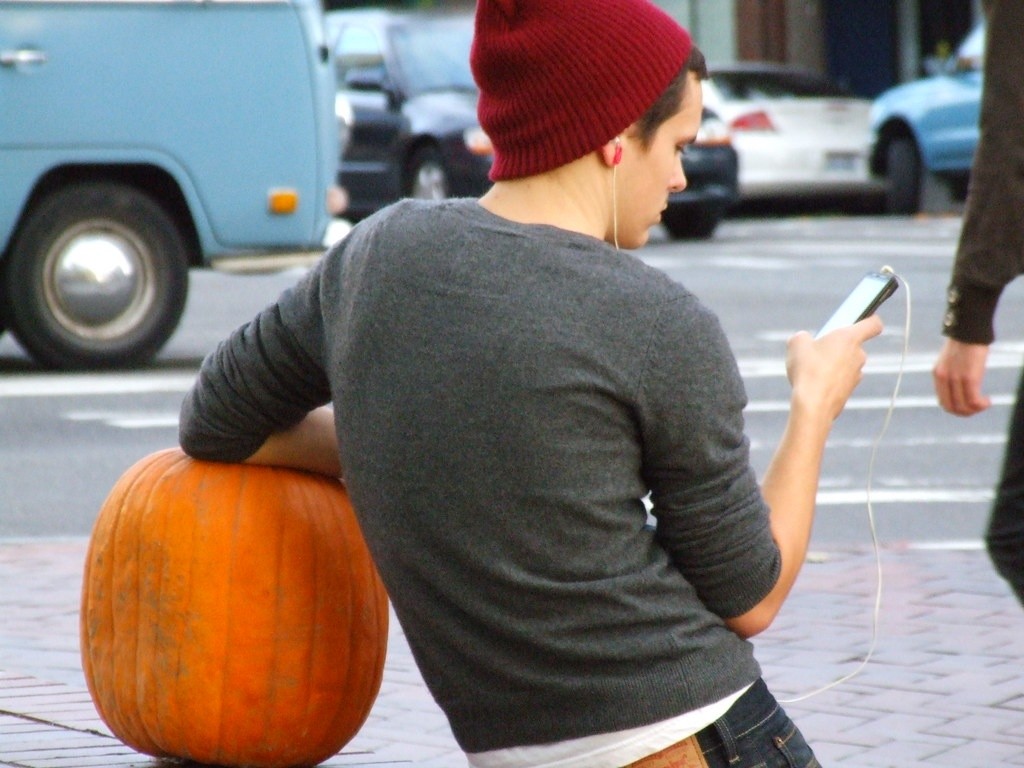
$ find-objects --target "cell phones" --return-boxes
[812,271,899,341]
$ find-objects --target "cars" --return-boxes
[1,1,340,376]
[695,63,874,217]
[871,12,987,215]
[340,20,740,238]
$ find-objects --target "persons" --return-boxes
[179,1,883,768]
[933,0,1024,606]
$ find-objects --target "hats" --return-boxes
[471,0,691,181]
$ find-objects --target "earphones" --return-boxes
[612,136,623,165]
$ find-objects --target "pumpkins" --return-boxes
[77,447,390,768]
